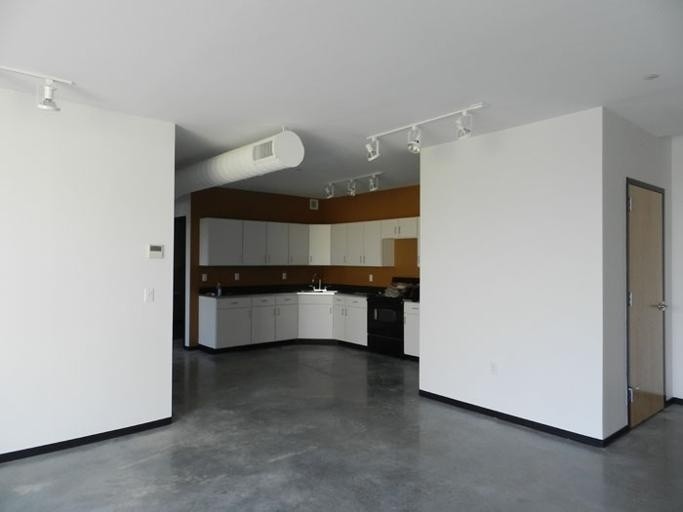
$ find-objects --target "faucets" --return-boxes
[312,271,328,291]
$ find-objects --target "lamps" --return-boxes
[324,174,380,198]
[364,107,473,162]
[35,77,61,112]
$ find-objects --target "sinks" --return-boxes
[296,290,339,295]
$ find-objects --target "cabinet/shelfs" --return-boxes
[402,301,419,356]
[252,293,297,344]
[346,220,395,267]
[242,221,289,267]
[289,223,330,266]
[330,295,367,346]
[298,295,333,339]
[330,223,346,266]
[197,296,251,350]
[199,218,242,266]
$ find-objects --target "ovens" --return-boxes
[366,297,404,357]
